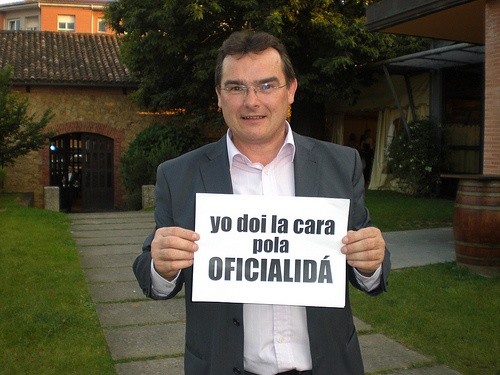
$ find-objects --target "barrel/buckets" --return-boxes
[453,179,500,268]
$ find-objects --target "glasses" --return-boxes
[218,82,286,94]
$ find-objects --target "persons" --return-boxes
[133,30,391,375]
